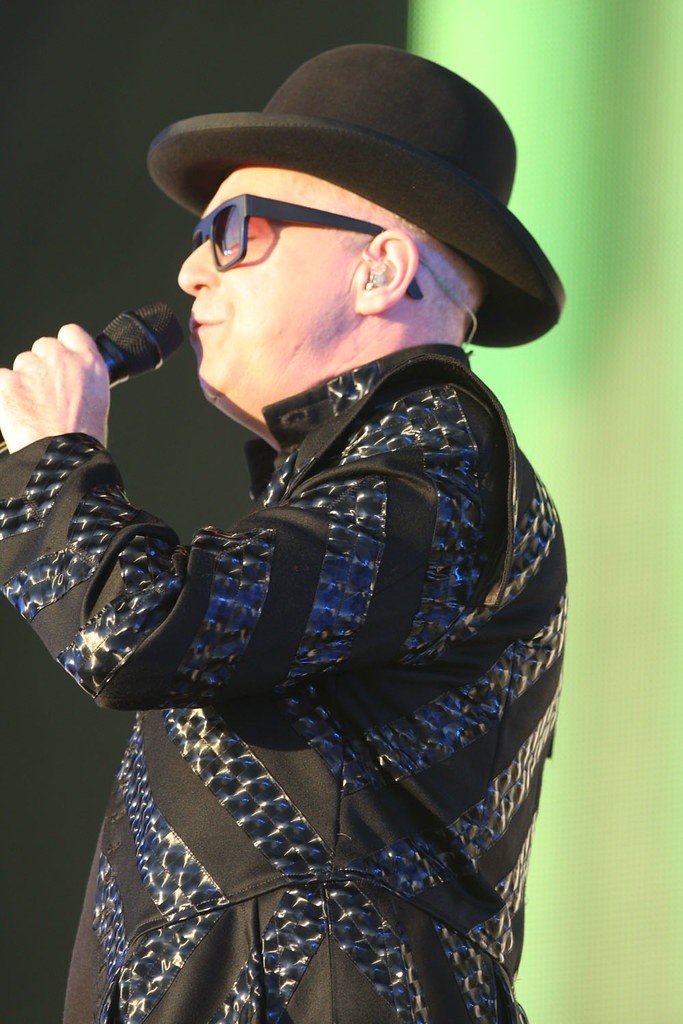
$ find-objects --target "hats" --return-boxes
[147,43,566,347]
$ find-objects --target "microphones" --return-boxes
[0,301,186,461]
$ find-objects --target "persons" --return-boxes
[0,44,571,1024]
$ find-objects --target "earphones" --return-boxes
[365,262,393,290]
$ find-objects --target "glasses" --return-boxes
[191,194,424,299]
